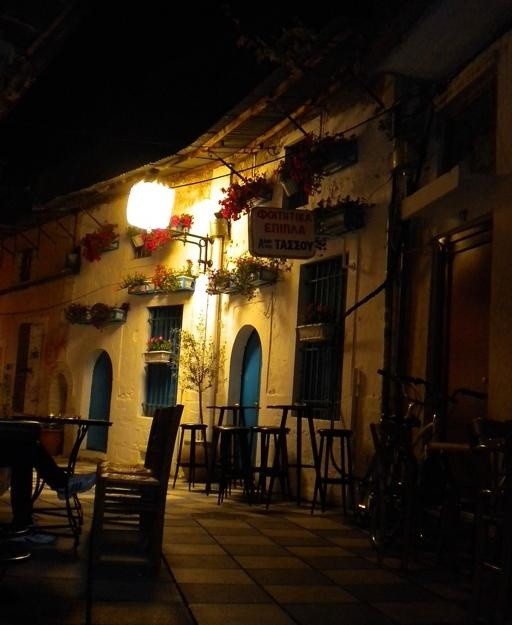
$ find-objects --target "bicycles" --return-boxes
[361,367,511,577]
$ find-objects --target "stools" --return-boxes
[173,401,352,512]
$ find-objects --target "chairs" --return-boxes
[12,405,181,577]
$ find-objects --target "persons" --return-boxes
[1,418,98,545]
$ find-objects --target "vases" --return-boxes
[321,213,366,236]
[296,324,330,344]
[142,351,172,365]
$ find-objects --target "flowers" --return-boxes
[211,173,272,219]
[297,301,335,322]
[206,257,276,292]
[125,262,194,293]
[66,300,126,327]
[80,223,116,261]
[145,335,172,353]
[280,130,328,196]
[313,193,375,212]
[128,215,193,249]
